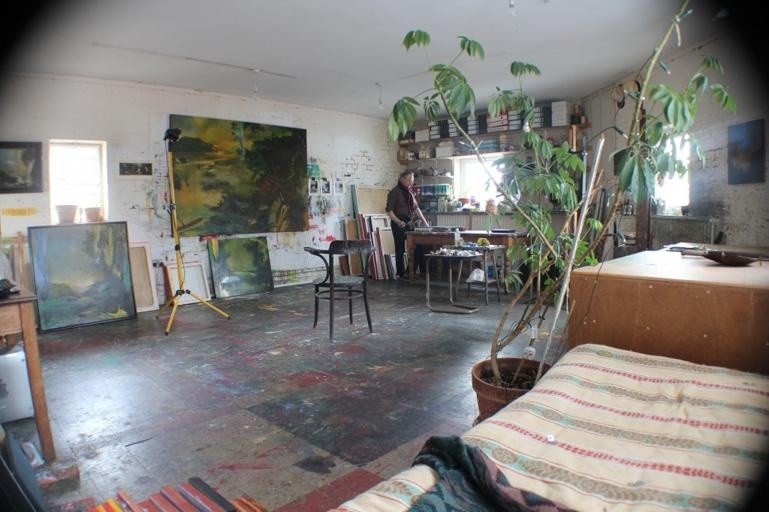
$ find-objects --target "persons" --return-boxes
[385,170,431,283]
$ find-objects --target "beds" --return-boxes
[328,342,769,512]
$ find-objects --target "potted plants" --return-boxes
[387,0,738,427]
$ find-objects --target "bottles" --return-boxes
[455,227,461,247]
[448,196,457,212]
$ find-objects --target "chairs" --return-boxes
[305,239,377,339]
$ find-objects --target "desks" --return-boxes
[424,254,483,314]
[404,230,533,292]
[567,241,769,374]
[455,246,504,305]
[0,285,56,460]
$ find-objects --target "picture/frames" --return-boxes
[14,221,211,331]
[345,184,408,280]
[0,141,43,194]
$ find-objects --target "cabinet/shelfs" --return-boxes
[397,125,592,164]
[413,174,454,197]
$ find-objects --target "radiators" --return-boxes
[437,214,514,230]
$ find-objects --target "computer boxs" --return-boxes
[0,346,35,423]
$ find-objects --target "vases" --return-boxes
[86,207,101,222]
[56,205,76,223]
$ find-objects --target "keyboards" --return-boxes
[0,279,19,294]
